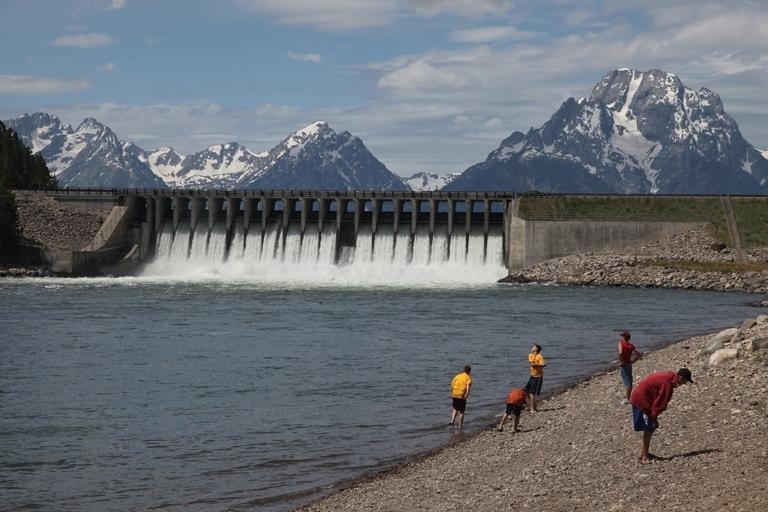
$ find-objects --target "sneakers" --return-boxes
[622,399,631,404]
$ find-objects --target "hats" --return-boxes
[679,368,694,384]
[620,331,631,339]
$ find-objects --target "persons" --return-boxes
[497,387,529,434]
[524,343,547,414]
[448,363,473,429]
[617,331,641,406]
[629,367,695,464]
